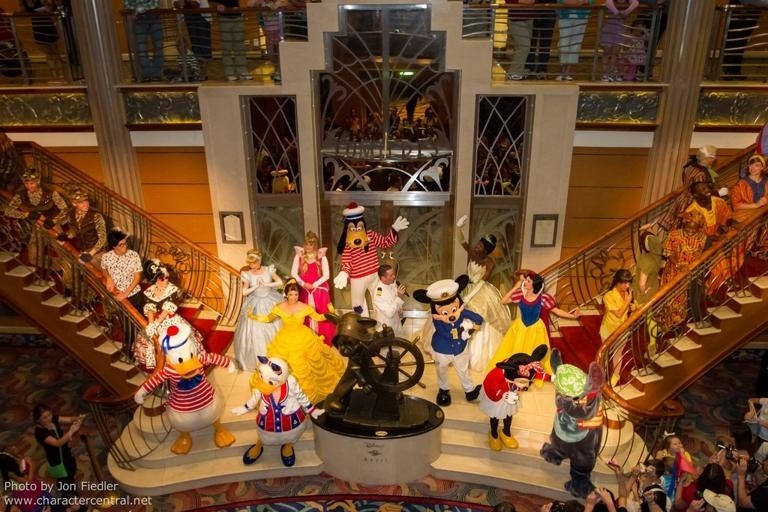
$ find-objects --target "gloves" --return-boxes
[28,211,39,220]
[81,253,92,261]
[259,278,280,289]
[57,233,67,242]
[456,215,468,244]
[244,280,258,296]
[462,279,484,303]
[44,218,54,229]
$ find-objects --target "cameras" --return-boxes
[608,460,619,467]
[737,456,760,473]
[725,443,735,459]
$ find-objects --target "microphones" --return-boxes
[396,280,410,297]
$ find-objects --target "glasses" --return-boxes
[118,242,127,249]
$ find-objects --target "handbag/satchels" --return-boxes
[46,464,68,480]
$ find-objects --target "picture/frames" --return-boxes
[218,211,247,244]
[530,213,558,247]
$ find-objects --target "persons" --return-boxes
[1,130,181,364]
[232,201,410,407]
[595,145,768,387]
[243,104,520,196]
[132,318,236,455]
[540,364,604,500]
[31,403,85,511]
[484,269,581,379]
[421,213,512,360]
[479,345,556,452]
[119,1,283,84]
[541,397,768,512]
[20,1,86,83]
[230,355,327,467]
[458,2,647,82]
[413,274,481,407]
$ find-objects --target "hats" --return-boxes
[703,489,736,512]
[70,189,89,202]
[22,170,42,184]
